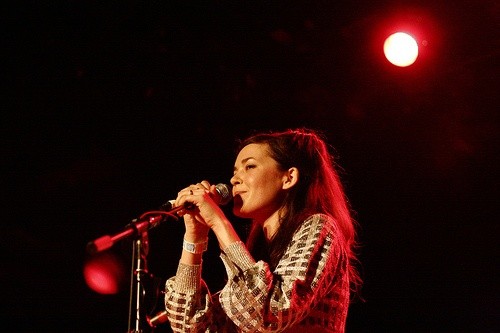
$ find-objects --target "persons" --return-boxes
[165,132,348,333]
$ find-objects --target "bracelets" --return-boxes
[182,238,209,253]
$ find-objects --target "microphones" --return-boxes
[160,183,232,212]
